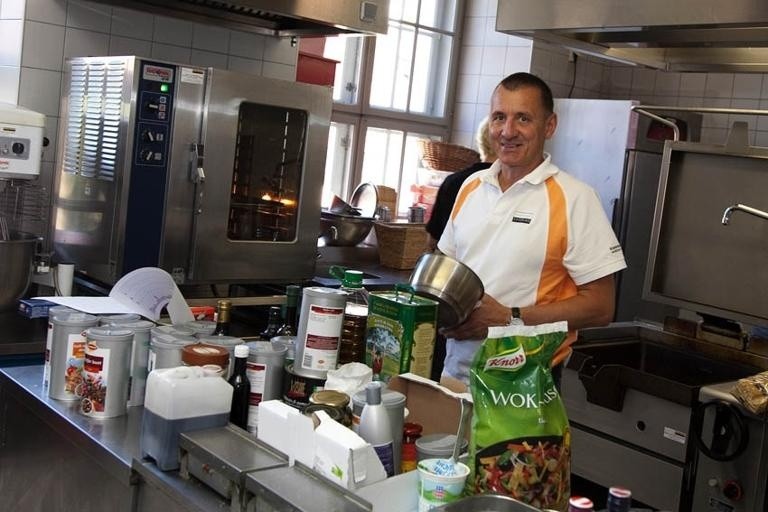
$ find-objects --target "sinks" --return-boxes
[575,320,766,389]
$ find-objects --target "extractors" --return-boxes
[92,0,390,35]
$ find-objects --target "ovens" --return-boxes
[46,56,334,286]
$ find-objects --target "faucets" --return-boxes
[721,203,767,228]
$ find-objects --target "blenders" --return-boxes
[0,97,50,321]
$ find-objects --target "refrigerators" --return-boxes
[544,96,703,325]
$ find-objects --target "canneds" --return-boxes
[399,422,423,474]
[309,388,354,427]
[285,365,327,411]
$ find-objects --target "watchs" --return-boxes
[507,307,525,327]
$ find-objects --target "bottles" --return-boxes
[606,487,632,512]
[276,285,300,336]
[227,344,251,430]
[259,307,284,341]
[567,495,594,512]
[359,382,394,476]
[212,298,236,337]
[339,270,369,363]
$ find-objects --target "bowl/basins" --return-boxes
[320,209,375,246]
[417,168,454,188]
[409,253,484,328]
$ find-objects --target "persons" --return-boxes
[424,117,499,380]
[436,70,629,395]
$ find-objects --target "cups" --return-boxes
[417,458,470,512]
[408,207,425,223]
[54,263,75,300]
[415,432,468,468]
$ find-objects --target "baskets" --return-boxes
[419,139,480,172]
[375,223,432,270]
